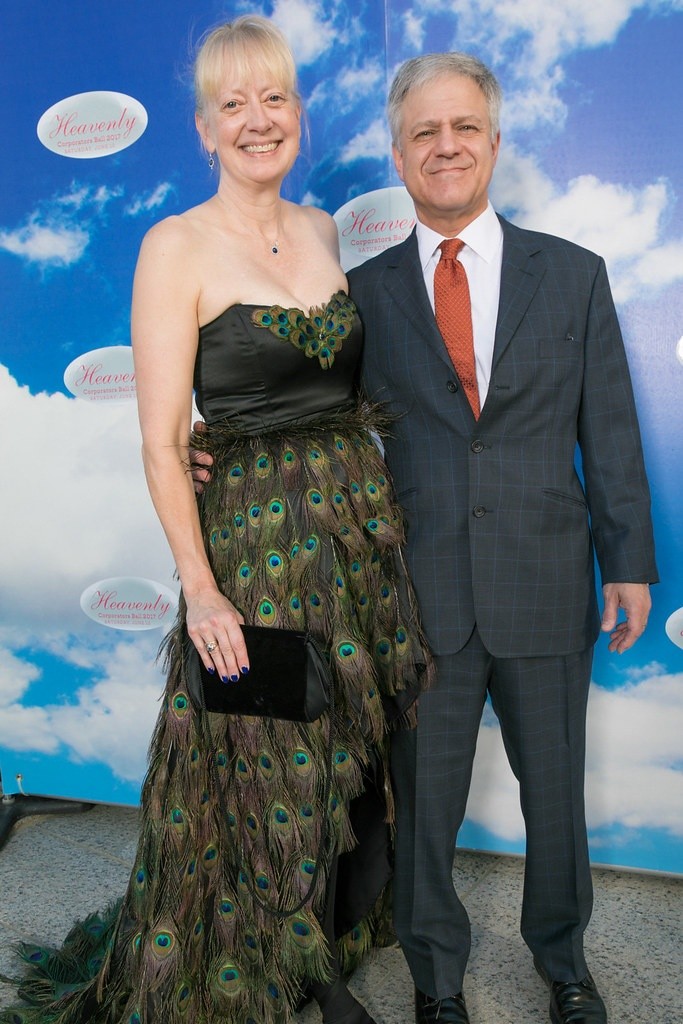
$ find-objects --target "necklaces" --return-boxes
[218,193,280,253]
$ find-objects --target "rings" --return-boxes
[204,641,219,653]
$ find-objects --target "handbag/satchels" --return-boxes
[199,622,336,918]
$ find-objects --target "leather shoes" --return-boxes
[415,982,470,1024]
[532,955,607,1024]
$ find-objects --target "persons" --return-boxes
[0,14,434,1024]
[190,52,659,1024]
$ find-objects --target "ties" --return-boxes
[434,239,480,421]
